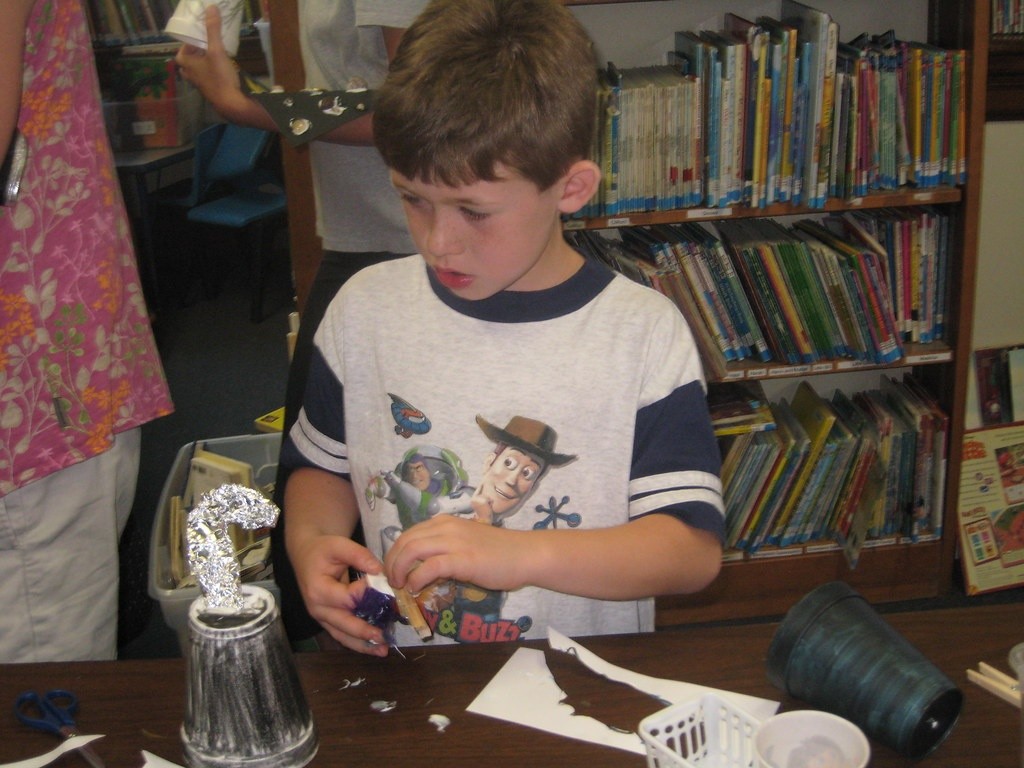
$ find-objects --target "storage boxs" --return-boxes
[146,429,288,642]
[125,57,202,150]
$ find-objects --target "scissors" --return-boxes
[13,689,105,768]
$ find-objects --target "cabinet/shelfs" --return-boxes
[557,0,995,630]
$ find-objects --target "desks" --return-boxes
[94,101,232,312]
[4,604,1024,766]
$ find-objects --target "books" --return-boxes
[167,443,277,590]
[548,0,972,566]
[83,0,271,102]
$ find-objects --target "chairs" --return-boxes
[189,119,285,320]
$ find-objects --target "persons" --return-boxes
[1,1,172,665]
[172,0,446,653]
[277,0,735,663]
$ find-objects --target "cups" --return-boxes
[754,710,874,766]
[765,577,966,764]
